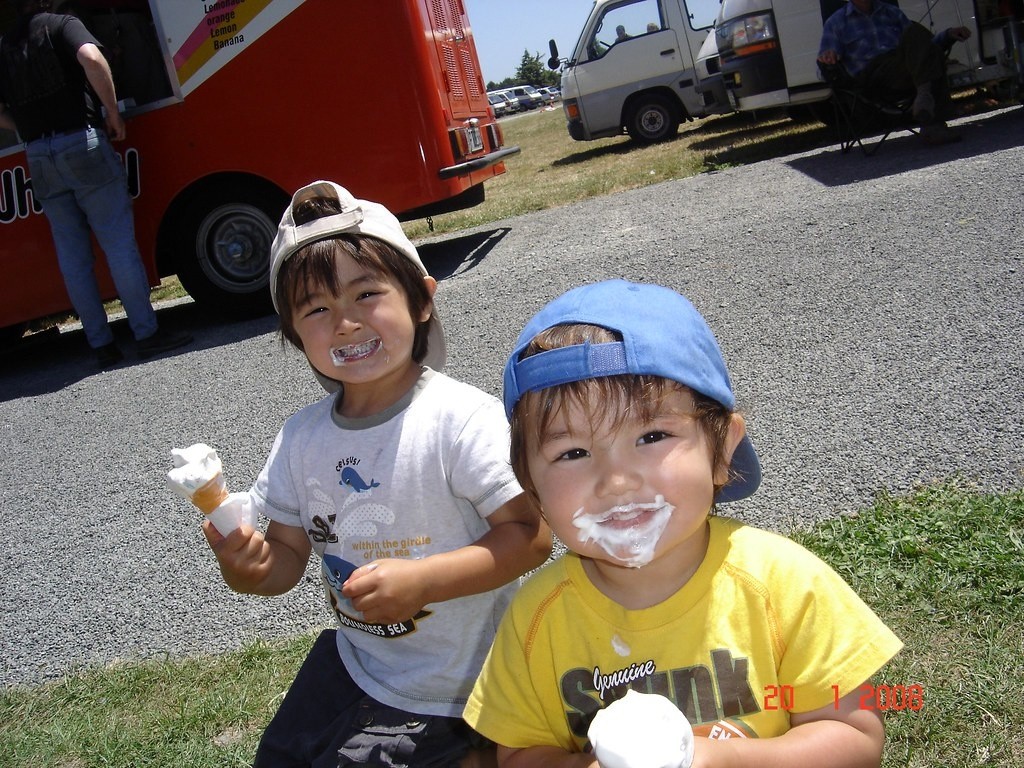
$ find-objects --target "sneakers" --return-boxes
[94,341,125,368]
[136,328,194,353]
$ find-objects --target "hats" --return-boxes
[502,279,761,501]
[268,181,448,394]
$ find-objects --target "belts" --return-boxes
[23,120,103,144]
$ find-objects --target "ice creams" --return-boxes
[587,689,695,768]
[165,442,230,514]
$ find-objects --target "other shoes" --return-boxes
[919,122,960,144]
[912,92,935,120]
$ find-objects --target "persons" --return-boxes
[615,25,634,43]
[647,25,659,33]
[200,178,555,768]
[460,276,908,768]
[0,1,196,371]
[816,0,972,145]
